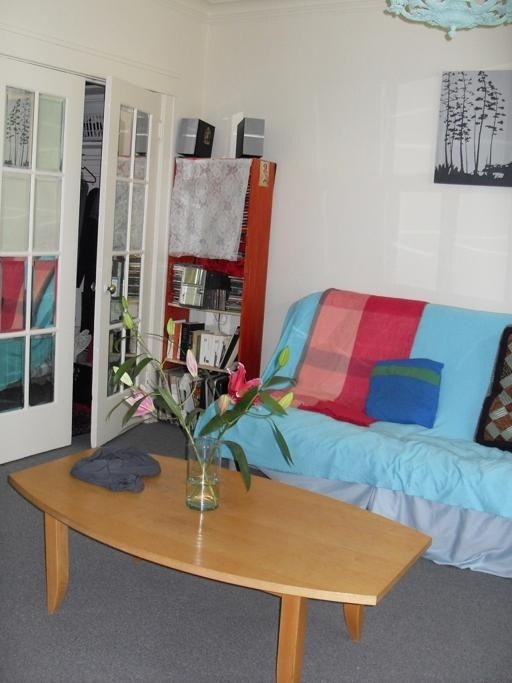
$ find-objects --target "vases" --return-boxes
[186,437,221,512]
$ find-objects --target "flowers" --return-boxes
[107,297,297,506]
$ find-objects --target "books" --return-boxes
[157,320,243,426]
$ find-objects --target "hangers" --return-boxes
[81,153,97,183]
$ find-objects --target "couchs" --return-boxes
[192,287,512,580]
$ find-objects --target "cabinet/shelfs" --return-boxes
[161,157,277,433]
